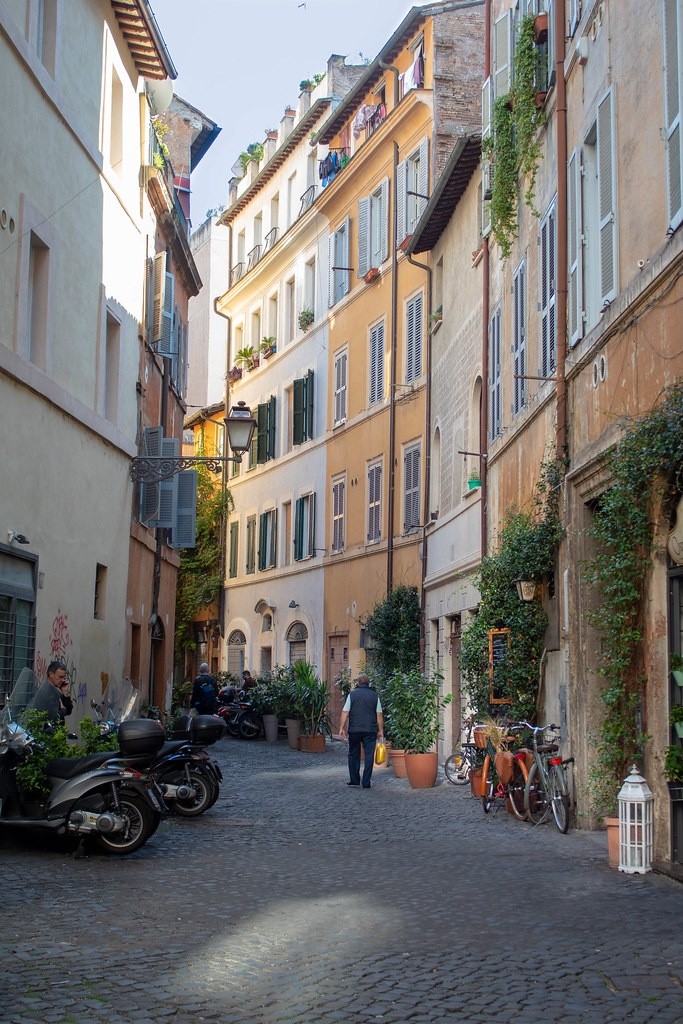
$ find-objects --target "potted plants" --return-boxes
[467,467,482,490]
[376,666,546,814]
[477,7,553,263]
[428,304,442,323]
[252,654,338,755]
[264,70,324,141]
[232,335,279,372]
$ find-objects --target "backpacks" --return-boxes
[199,682,215,700]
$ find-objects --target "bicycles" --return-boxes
[445,712,577,834]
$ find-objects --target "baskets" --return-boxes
[474,728,490,748]
[537,735,561,757]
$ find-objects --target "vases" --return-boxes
[364,265,379,284]
[602,815,649,871]
[399,234,411,251]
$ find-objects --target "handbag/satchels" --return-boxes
[375,736,386,764]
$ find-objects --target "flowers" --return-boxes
[221,366,243,386]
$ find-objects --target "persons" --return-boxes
[236,670,258,702]
[190,663,220,716]
[32,661,73,737]
[339,674,384,789]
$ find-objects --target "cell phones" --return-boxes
[60,681,69,687]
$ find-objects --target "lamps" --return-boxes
[13,534,30,545]
[512,570,546,608]
[288,600,299,609]
[128,400,261,487]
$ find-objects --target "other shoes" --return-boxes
[347,781,359,785]
[363,786,371,788]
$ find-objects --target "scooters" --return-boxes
[215,686,265,738]
[0,665,228,856]
